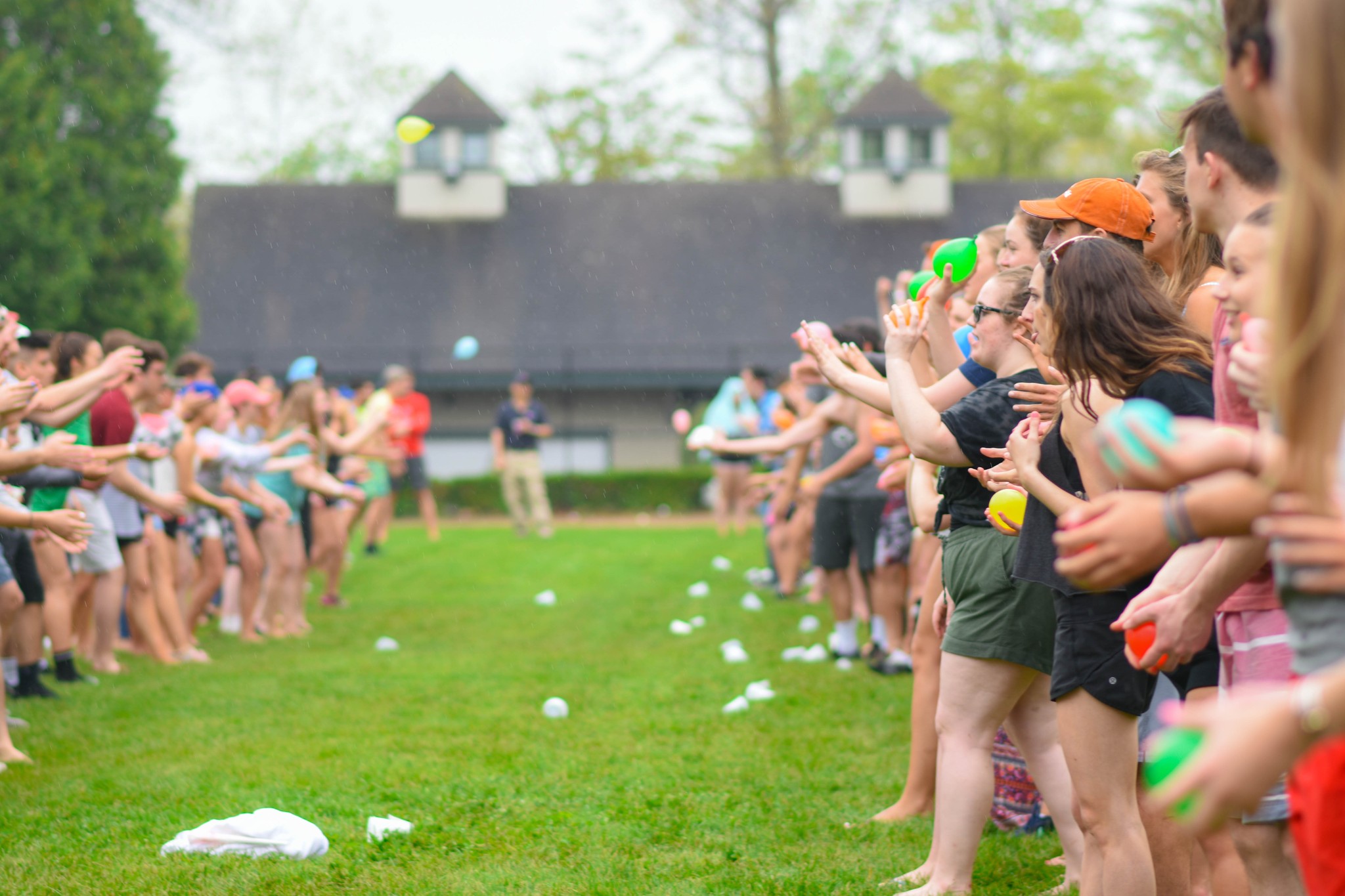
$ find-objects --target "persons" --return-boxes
[883,266,1085,896]
[0,305,442,772]
[490,375,554,540]
[685,0,1345,896]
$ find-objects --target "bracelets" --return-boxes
[1165,481,1201,549]
[126,441,137,456]
[1291,679,1329,743]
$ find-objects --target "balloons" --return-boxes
[887,296,929,328]
[395,115,435,144]
[917,274,952,314]
[450,335,480,362]
[989,488,1028,530]
[673,408,691,435]
[907,270,935,300]
[1101,399,1180,474]
[283,355,317,384]
[772,407,796,429]
[1141,726,1205,820]
[1124,620,1168,669]
[931,234,978,283]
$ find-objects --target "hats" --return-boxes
[225,377,271,407]
[1019,177,1157,244]
[0,304,31,339]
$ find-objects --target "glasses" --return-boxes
[1047,235,1104,265]
[971,304,1022,323]
[1170,145,1187,160]
